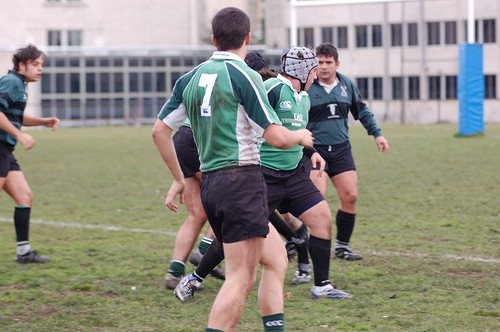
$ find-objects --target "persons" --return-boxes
[152,7,354,332]
[285,43,389,261]
[0,45,61,264]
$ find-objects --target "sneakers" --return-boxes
[291,262,312,283]
[165,271,186,288]
[310,284,353,300]
[284,239,298,263]
[14,250,50,264]
[188,247,225,280]
[173,273,205,304]
[334,244,362,261]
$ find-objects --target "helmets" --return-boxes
[280,47,320,89]
